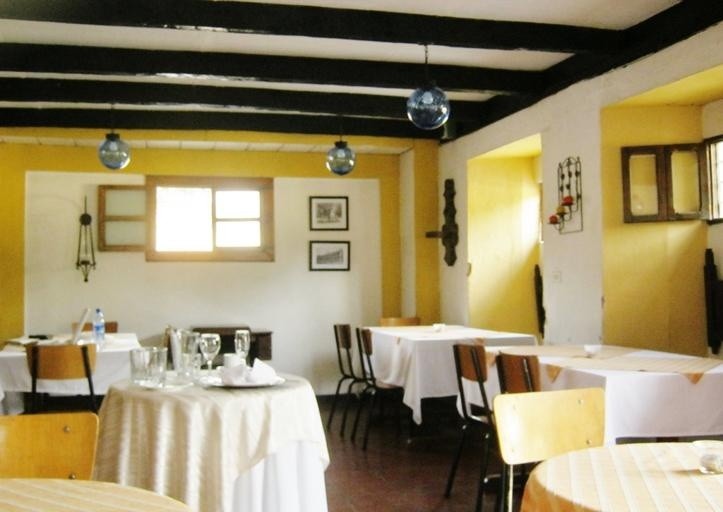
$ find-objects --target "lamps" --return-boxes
[404,41,451,132]
[325,112,358,177]
[99,97,131,172]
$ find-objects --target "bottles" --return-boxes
[92,308,105,343]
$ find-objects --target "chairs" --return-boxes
[23,341,100,412]
[0,409,100,480]
[70,319,120,336]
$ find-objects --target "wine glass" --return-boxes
[200,333,222,371]
[234,329,251,362]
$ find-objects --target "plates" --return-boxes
[200,372,286,388]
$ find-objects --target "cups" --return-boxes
[144,346,168,387]
[131,348,154,386]
[170,332,199,375]
[223,353,243,375]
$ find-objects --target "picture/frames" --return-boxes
[309,240,350,272]
[308,194,349,232]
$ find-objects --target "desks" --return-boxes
[191,325,273,365]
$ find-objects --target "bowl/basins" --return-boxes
[584,344,602,355]
[692,438,723,474]
[433,323,444,332]
